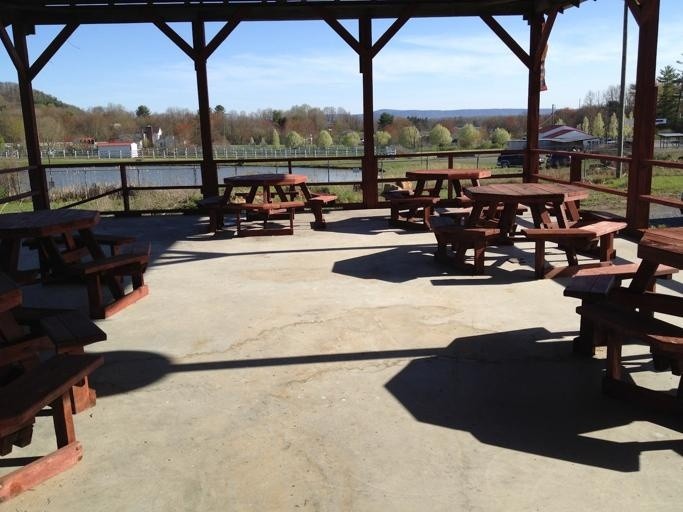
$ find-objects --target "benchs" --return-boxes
[385,188,683,398]
[195,192,337,237]
[0,235,152,502]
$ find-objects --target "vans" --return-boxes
[496,139,580,167]
[656,119,669,125]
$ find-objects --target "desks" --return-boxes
[0,210,124,299]
[223,174,317,218]
[406,169,492,222]
